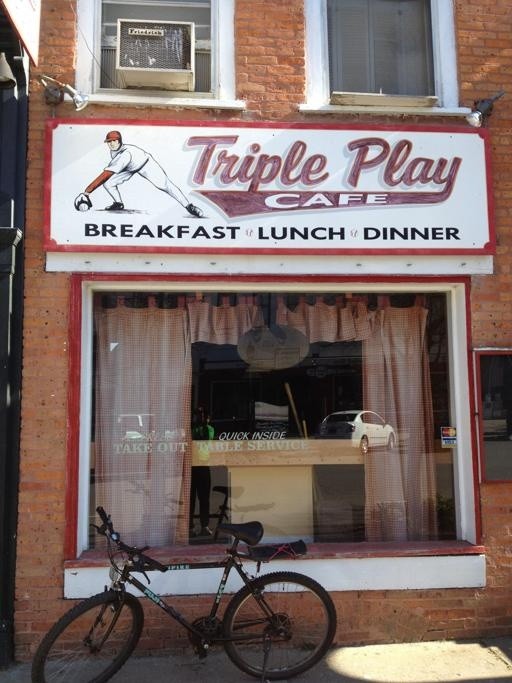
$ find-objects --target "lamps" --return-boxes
[464,90,506,129]
[35,72,90,111]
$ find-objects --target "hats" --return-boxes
[106,131,123,142]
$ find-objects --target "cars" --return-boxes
[320,410,396,454]
[115,414,157,427]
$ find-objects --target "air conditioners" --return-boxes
[115,18,196,93]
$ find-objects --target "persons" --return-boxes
[75,130,204,218]
[186,405,216,537]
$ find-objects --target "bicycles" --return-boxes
[32,507,335,683]
[126,479,285,543]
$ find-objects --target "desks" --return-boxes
[88,437,452,543]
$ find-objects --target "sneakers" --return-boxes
[186,204,203,218]
[105,203,123,210]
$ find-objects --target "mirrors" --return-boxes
[473,347,512,484]
